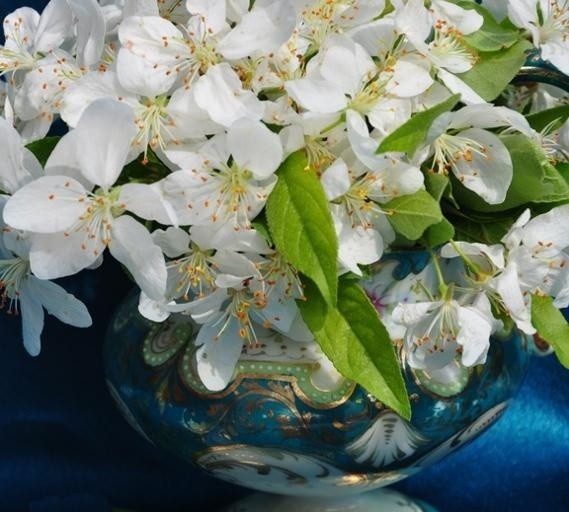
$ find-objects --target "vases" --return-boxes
[97,246,530,499]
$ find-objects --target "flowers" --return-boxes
[0,0,569,420]
